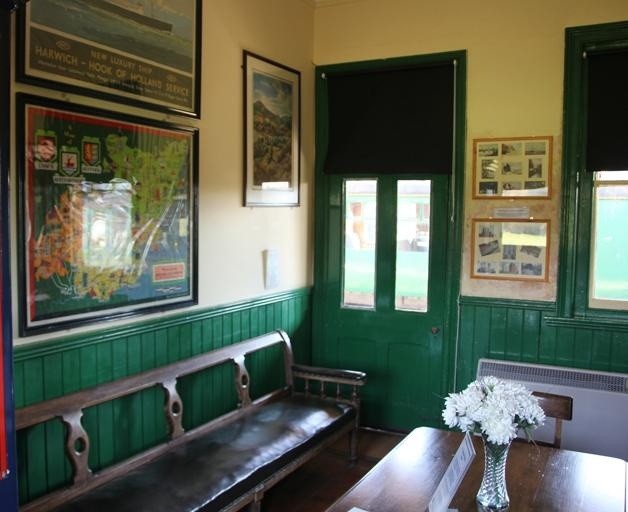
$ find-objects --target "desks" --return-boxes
[315,426,626,512]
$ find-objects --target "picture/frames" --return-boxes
[240,50,301,210]
[471,136,552,201]
[14,0,204,123]
[470,217,551,284]
[12,91,199,336]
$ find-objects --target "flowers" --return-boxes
[440,375,544,446]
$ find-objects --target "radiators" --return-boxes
[477,359,628,462]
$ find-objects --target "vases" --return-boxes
[482,430,514,511]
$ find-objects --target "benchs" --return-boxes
[11,323,369,512]
